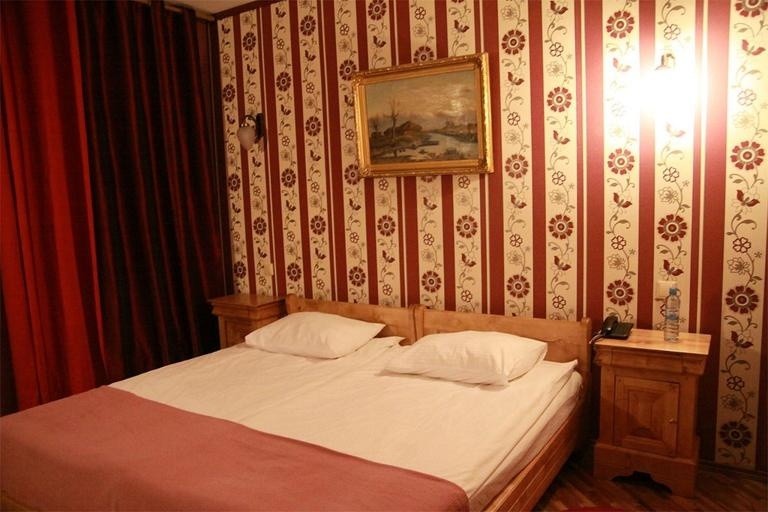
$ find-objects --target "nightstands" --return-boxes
[588,328,711,496]
[207,294,282,348]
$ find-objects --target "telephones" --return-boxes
[602,315,634,339]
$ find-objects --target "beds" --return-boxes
[0,297,592,511]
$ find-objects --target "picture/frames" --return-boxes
[349,52,494,178]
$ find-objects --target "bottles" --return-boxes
[664,288,680,343]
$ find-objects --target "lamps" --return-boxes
[651,55,685,117]
[236,112,264,151]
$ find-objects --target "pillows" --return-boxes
[383,331,548,386]
[244,312,385,359]
